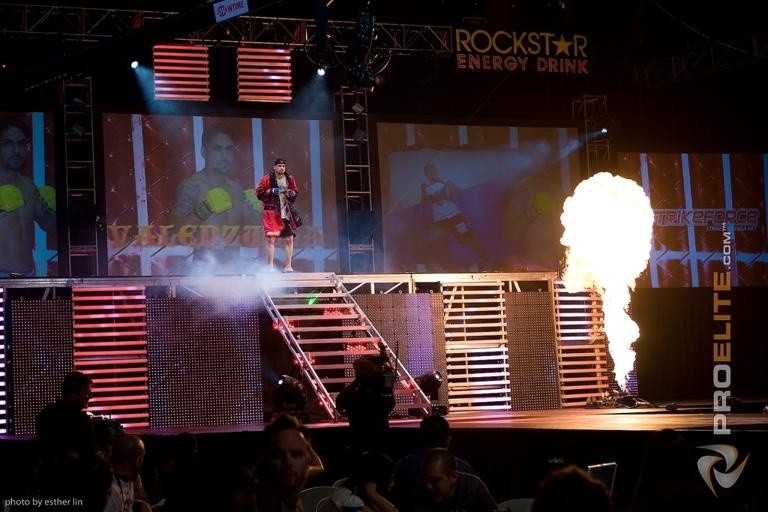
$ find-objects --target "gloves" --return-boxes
[0,184,25,215]
[194,187,232,221]
[287,190,296,197]
[242,189,262,214]
[272,187,279,195]
[38,186,56,216]
[532,191,552,216]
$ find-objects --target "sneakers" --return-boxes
[284,266,293,272]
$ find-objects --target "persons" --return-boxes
[501,151,562,265]
[174,123,263,265]
[420,164,475,273]
[255,158,304,272]
[0,115,57,278]
[31,355,691,512]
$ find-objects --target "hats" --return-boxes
[275,159,286,165]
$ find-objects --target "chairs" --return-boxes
[279,461,621,511]
